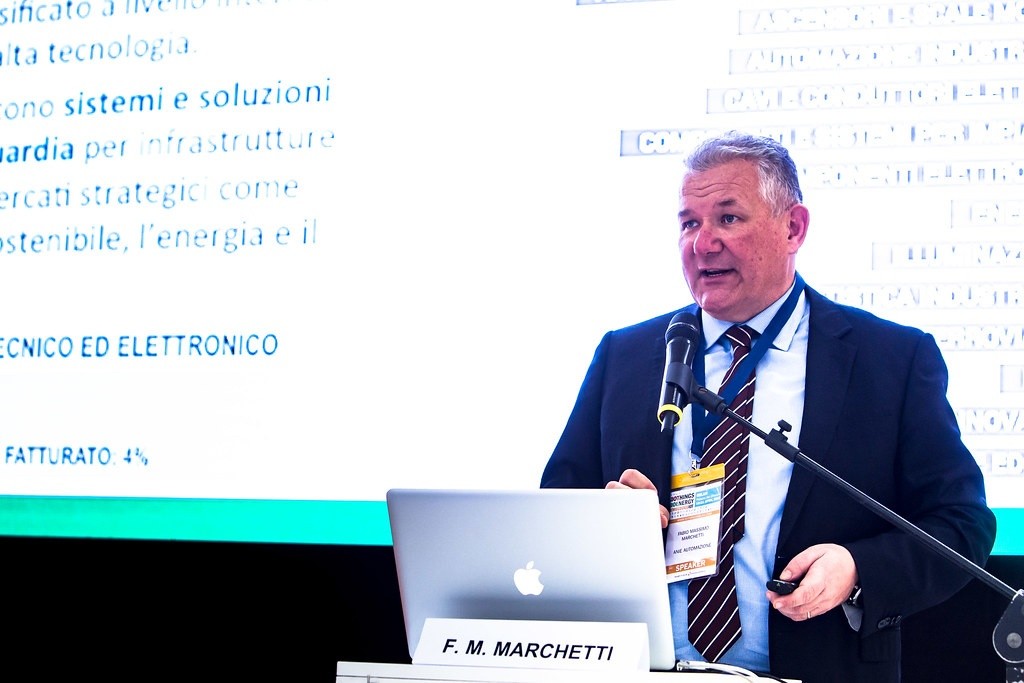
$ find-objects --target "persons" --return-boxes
[540,130,996,683]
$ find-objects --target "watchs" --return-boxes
[845,584,862,608]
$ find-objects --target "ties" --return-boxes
[688,327,764,664]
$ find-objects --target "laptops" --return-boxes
[386,488,679,672]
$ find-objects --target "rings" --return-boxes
[807,612,811,619]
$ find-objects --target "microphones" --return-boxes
[658,311,701,432]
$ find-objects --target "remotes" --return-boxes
[765,579,797,595]
